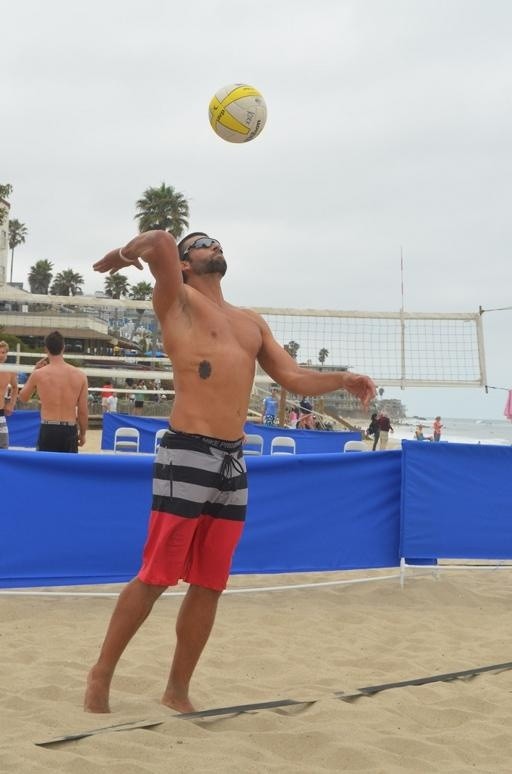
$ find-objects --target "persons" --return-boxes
[86,230,376,714]
[19,331,88,453]
[149,379,160,406]
[102,381,117,412]
[0,341,18,449]
[434,416,443,441]
[114,345,120,356]
[369,412,394,451]
[414,425,432,441]
[125,378,147,416]
[290,395,312,429]
[261,389,280,426]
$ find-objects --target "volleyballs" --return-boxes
[208,82,267,143]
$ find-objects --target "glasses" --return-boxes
[183,238,221,256]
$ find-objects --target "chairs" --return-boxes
[114,426,297,458]
[343,441,369,452]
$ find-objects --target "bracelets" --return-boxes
[119,248,136,263]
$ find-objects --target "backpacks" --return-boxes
[368,422,375,434]
